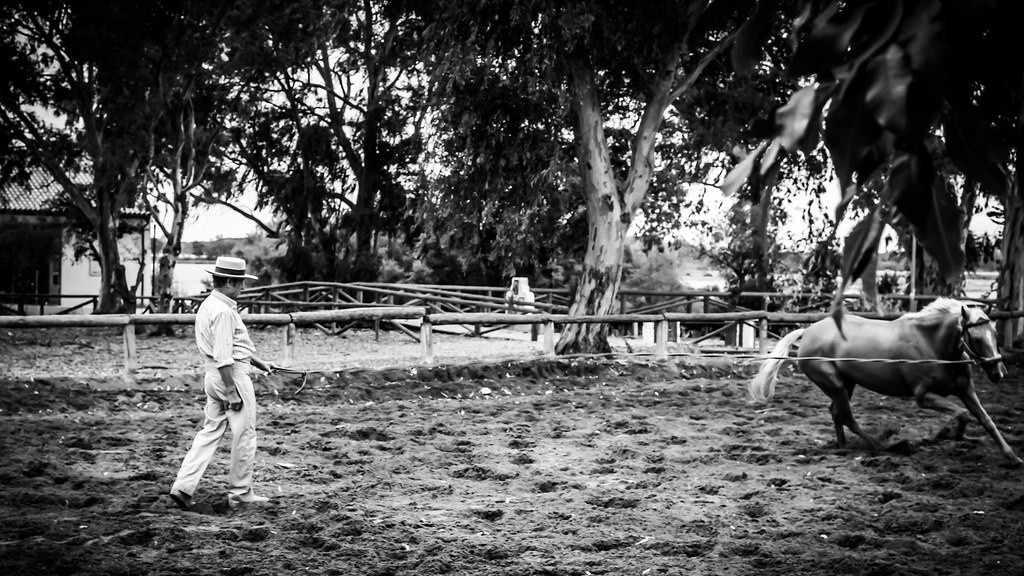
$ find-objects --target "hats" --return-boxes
[205,257,259,280]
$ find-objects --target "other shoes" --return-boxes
[228,493,269,509]
[170,487,197,509]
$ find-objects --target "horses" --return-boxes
[747,297,1024,465]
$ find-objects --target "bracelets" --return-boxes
[227,389,237,396]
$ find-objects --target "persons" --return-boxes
[169,256,277,510]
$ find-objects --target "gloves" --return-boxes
[227,386,242,411]
